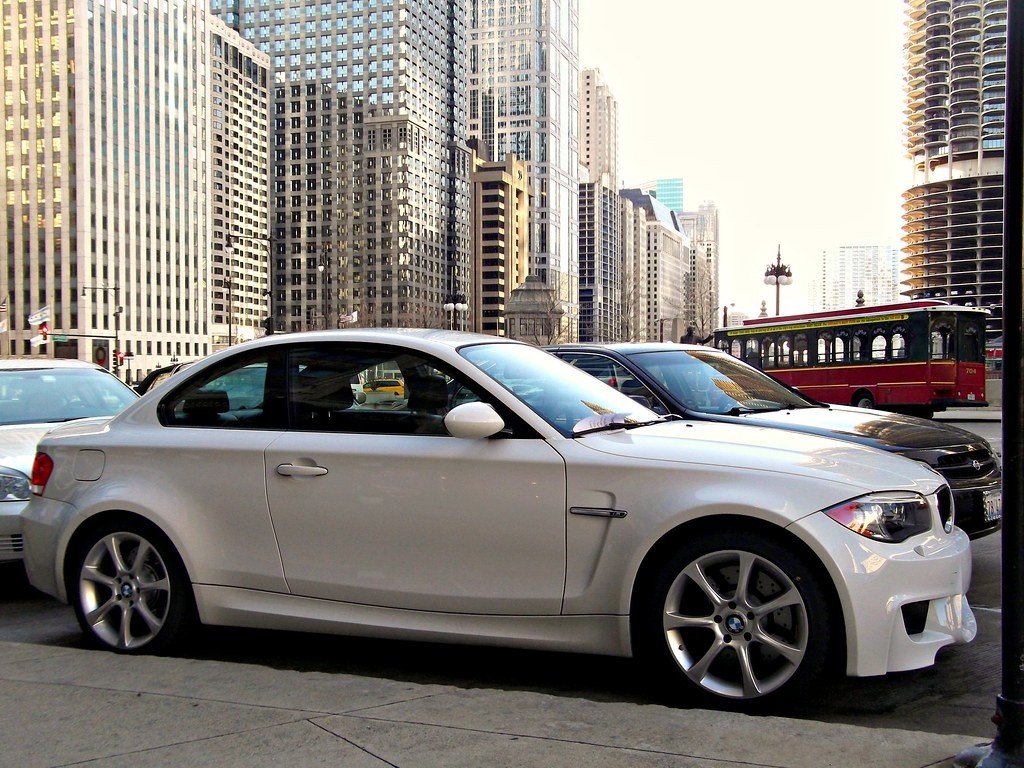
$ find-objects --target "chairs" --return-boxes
[1,399,25,422]
[621,380,653,406]
[184,390,237,426]
[298,368,353,429]
[398,375,449,436]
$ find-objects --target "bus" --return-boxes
[694,298,990,419]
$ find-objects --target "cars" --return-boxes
[537,342,1002,542]
[1,358,143,564]
[133,362,192,398]
[19,327,980,706]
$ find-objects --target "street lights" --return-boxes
[443,276,468,331]
[225,232,279,337]
[81,286,121,378]
[763,244,792,316]
[317,241,328,330]
[221,278,232,347]
